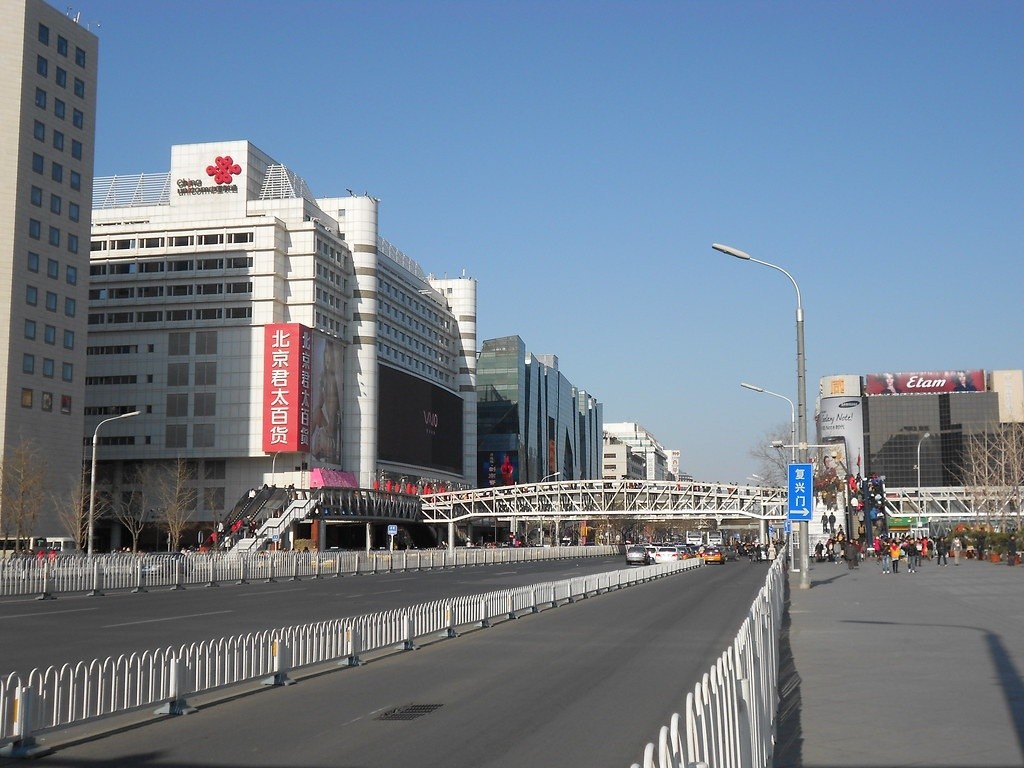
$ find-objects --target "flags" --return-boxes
[857,454,860,467]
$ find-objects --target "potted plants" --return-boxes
[945,531,955,557]
[1014,540,1024,565]
[984,531,1009,563]
[970,530,987,560]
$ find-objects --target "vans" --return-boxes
[46,537,77,552]
[561,535,573,546]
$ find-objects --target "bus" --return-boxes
[685,530,724,546]
[0,536,47,558]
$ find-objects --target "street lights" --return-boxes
[541,471,561,482]
[87,411,143,558]
[914,432,932,538]
[740,383,801,573]
[712,242,809,589]
[746,474,772,486]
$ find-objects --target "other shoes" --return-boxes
[938,565,940,566]
[908,569,911,572]
[835,562,837,564]
[912,570,914,573]
[945,565,947,567]
[954,564,958,566]
[886,571,889,574]
[839,561,842,564]
[883,571,885,574]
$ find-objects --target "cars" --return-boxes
[625,541,740,565]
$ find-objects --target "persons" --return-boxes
[748,541,785,565]
[823,455,836,476]
[813,474,1016,574]
[431,478,788,503]
[880,373,902,394]
[197,483,309,553]
[11,547,195,558]
[951,371,976,392]
